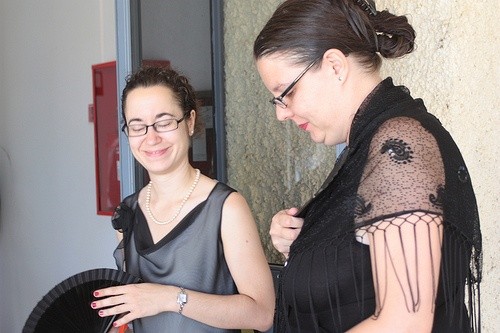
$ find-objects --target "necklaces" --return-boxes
[146,169,200,225]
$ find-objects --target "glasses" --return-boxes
[268,57,323,109]
[121,113,188,138]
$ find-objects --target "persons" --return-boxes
[252,0,483,333]
[92,66,276,333]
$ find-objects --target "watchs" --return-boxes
[177,286,187,314]
